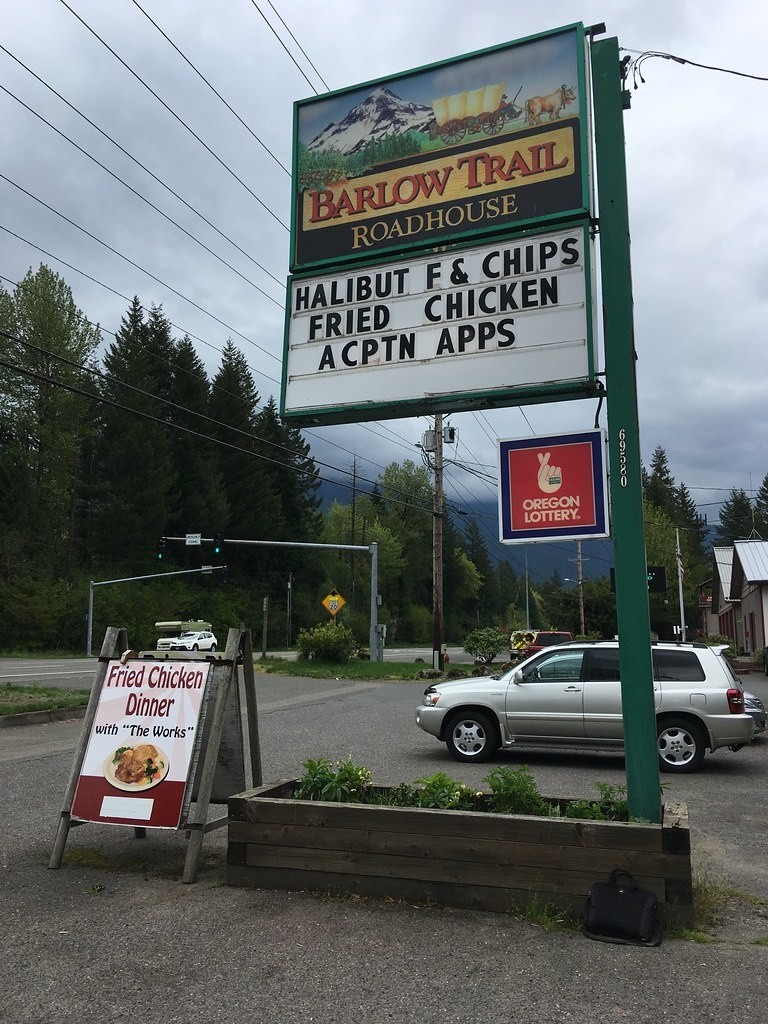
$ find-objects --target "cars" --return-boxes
[738,690,768,736]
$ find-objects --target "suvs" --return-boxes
[412,638,755,775]
[171,632,217,653]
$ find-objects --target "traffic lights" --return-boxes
[157,538,166,560]
[214,533,224,558]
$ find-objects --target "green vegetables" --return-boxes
[113,747,133,764]
[138,758,164,785]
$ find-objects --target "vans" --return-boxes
[153,620,213,650]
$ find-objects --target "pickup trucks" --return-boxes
[523,632,576,662]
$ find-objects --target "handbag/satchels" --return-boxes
[585,869,664,947]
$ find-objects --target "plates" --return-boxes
[103,743,169,792]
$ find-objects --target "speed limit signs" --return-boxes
[329,602,339,611]
